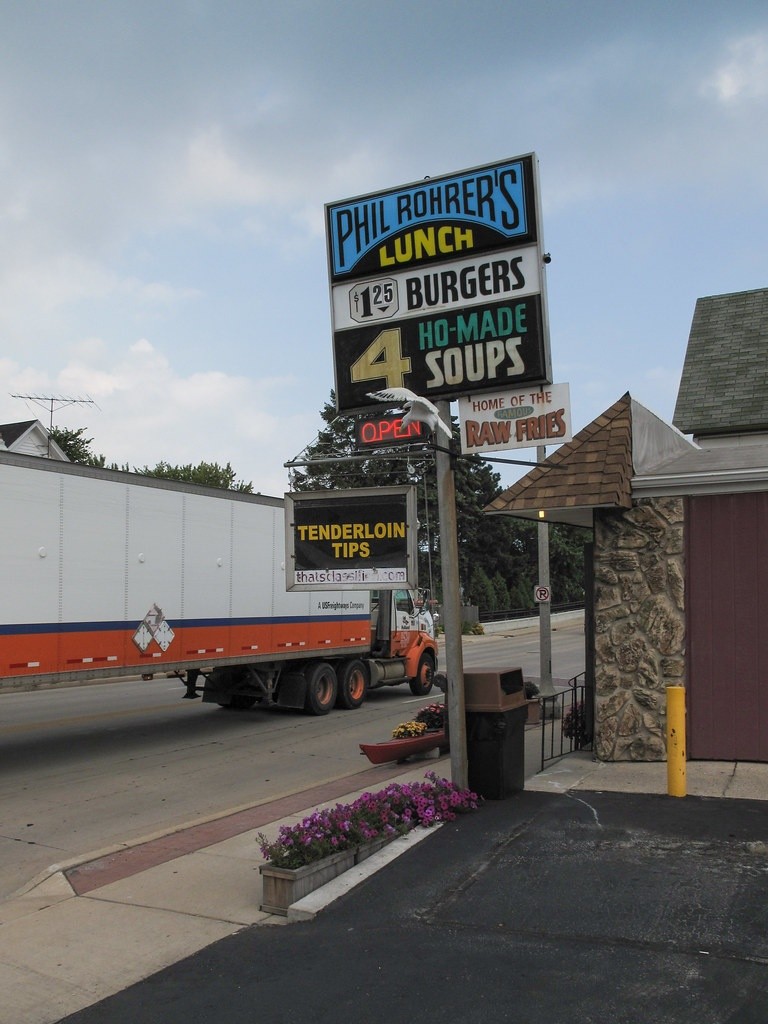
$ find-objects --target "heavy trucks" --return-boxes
[1,448,445,720]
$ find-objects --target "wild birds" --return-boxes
[364,387,454,440]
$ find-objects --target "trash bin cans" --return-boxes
[443,664,531,800]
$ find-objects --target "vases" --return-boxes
[259,831,404,917]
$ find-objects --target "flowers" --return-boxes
[411,702,445,729]
[254,769,488,868]
[392,720,428,740]
